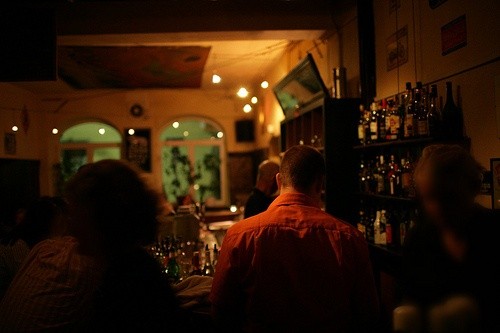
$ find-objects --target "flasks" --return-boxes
[334,67,347,99]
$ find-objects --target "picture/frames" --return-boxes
[490,158,500,209]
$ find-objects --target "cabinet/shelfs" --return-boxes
[280,99,469,249]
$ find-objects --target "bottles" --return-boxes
[155,204,221,284]
[357,80,458,146]
[358,147,422,199]
[356,204,422,253]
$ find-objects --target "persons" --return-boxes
[384,144,500,333]
[0,160,178,333]
[206,144,386,333]
[244,159,281,218]
